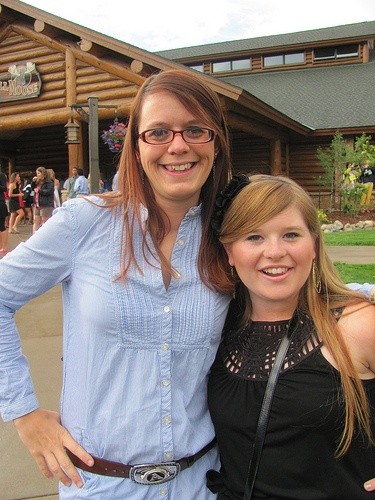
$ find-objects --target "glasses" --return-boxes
[137,128,215,144]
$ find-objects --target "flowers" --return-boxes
[101,122,127,152]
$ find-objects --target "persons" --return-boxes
[344,158,375,214]
[0,156,122,254]
[208,173,375,500]
[0,68,375,500]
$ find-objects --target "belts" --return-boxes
[65,435,217,485]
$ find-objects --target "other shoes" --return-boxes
[0,249,9,253]
[12,225,20,233]
[8,230,16,234]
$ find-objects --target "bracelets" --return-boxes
[368,287,375,306]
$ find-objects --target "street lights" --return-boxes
[64,104,117,193]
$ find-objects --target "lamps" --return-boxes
[64,104,90,144]
